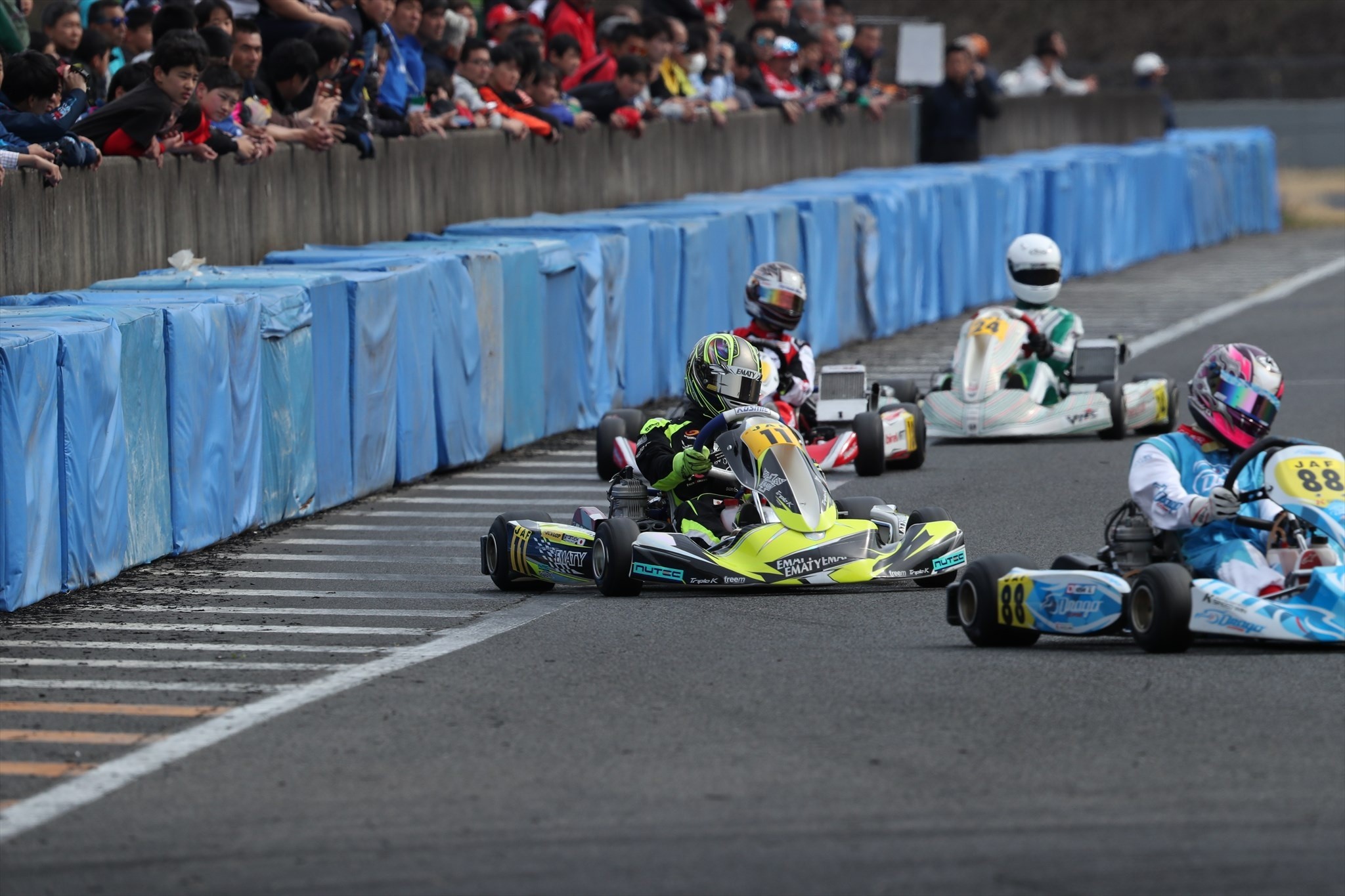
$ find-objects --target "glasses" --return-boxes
[106,16,130,28]
[474,58,494,69]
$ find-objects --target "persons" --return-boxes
[1128,342,1337,598]
[936,233,1084,409]
[1133,53,1179,132]
[634,334,763,549]
[0,0,1097,188]
[718,261,816,430]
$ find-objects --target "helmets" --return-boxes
[684,330,763,422]
[744,262,807,330]
[1188,341,1285,452]
[1005,233,1064,304]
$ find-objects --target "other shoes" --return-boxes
[940,378,952,390]
[734,503,761,528]
[1005,370,1027,390]
[762,402,779,413]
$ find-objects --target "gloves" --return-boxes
[674,442,712,477]
[777,367,795,395]
[1190,486,1241,527]
[1027,331,1053,359]
